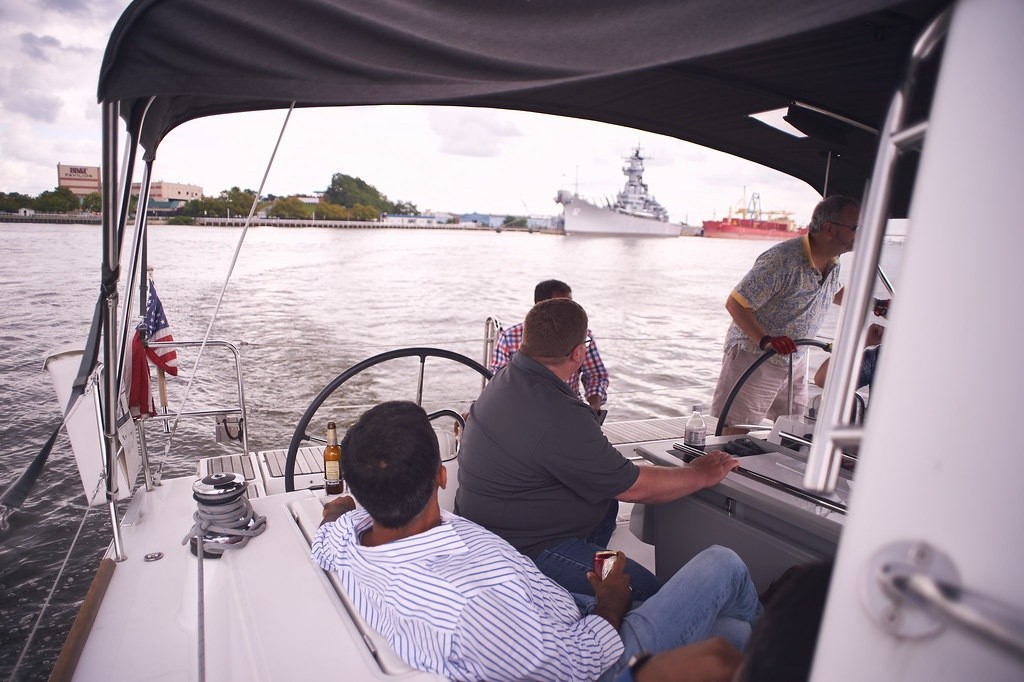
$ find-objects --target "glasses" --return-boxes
[567,334,592,359]
[829,220,856,231]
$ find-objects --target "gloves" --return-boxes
[874,298,891,319]
[759,335,798,354]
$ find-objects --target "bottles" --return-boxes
[683,405,706,463]
[324,422,343,495]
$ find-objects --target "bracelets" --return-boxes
[628,653,652,672]
[759,335,771,351]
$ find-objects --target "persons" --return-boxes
[453,298,741,600]
[613,554,833,682]
[310,400,763,682]
[491,279,609,414]
[815,323,886,394]
[710,195,893,436]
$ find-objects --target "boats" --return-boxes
[554,142,683,237]
[702,191,810,241]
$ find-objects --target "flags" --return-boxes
[124,279,178,419]
